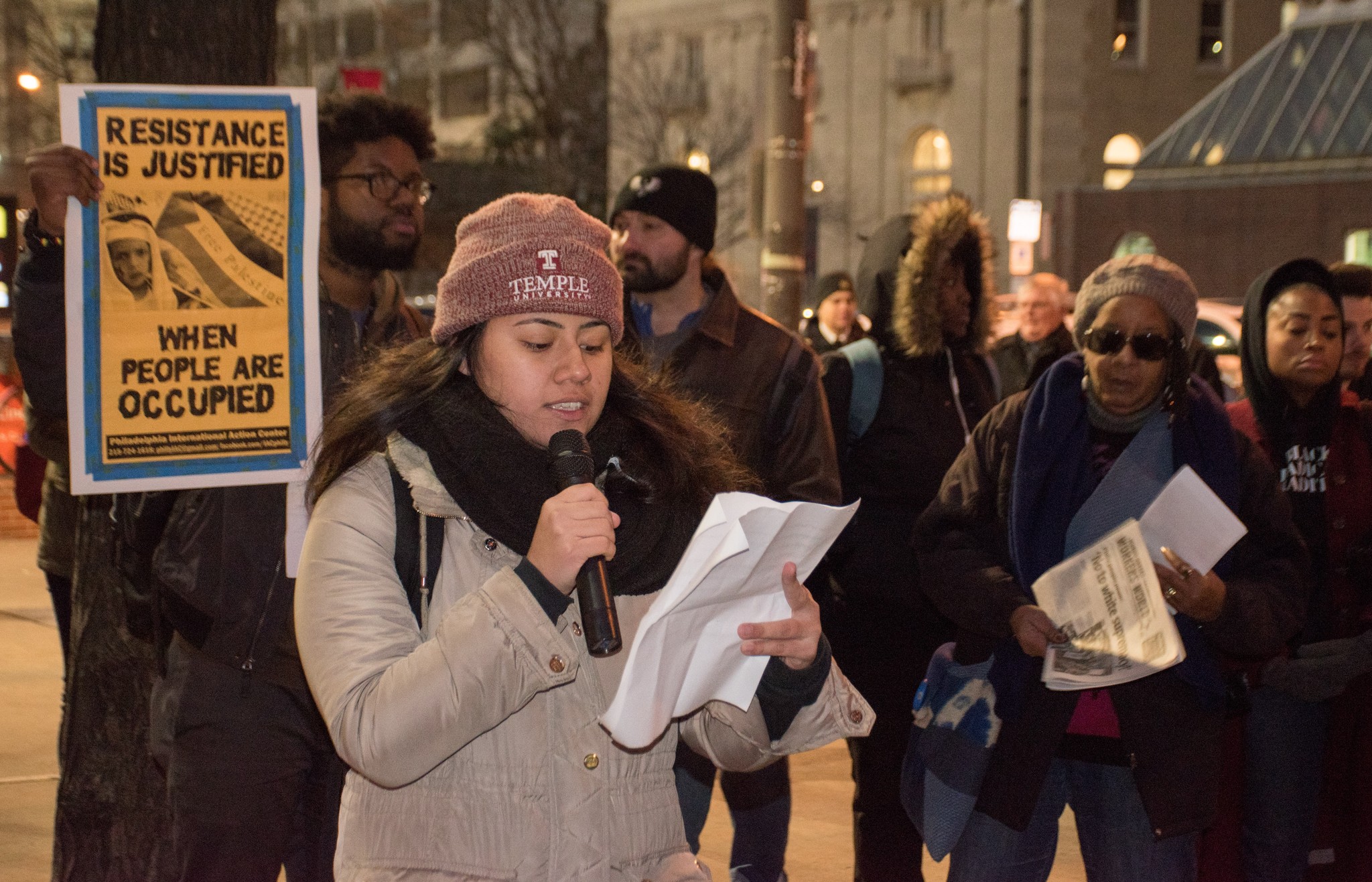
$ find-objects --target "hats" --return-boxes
[431,191,624,349]
[816,271,853,312]
[609,164,717,257]
[1072,255,1197,357]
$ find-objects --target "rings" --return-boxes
[1164,587,1177,598]
[1181,565,1194,579]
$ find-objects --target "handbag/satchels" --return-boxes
[14,436,50,525]
[900,642,1003,863]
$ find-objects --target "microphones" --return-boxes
[548,429,622,655]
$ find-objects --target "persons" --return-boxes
[605,162,841,882]
[293,192,880,882]
[9,82,437,882]
[799,192,1372,882]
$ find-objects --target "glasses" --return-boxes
[1084,328,1173,362]
[335,171,437,202]
